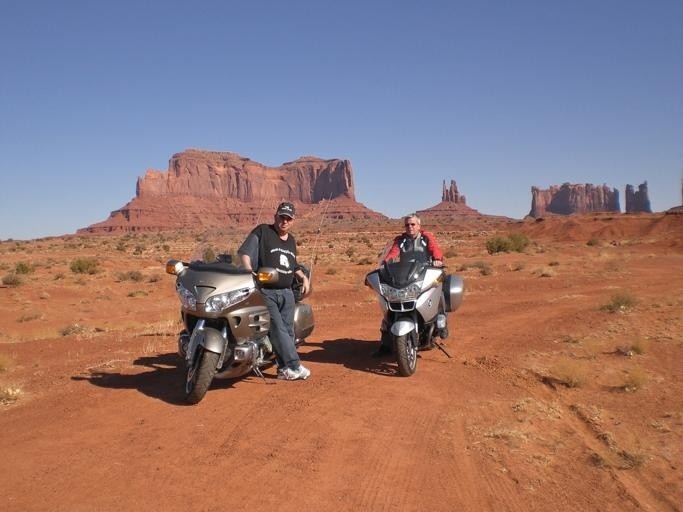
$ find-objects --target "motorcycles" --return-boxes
[363,249,466,376]
[168,193,339,404]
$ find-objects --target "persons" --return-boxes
[371,214,449,360]
[237,201,312,381]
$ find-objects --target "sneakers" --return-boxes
[440,327,448,339]
[277,364,311,380]
[372,349,391,357]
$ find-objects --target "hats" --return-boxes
[278,202,296,218]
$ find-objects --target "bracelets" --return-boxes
[301,274,307,280]
